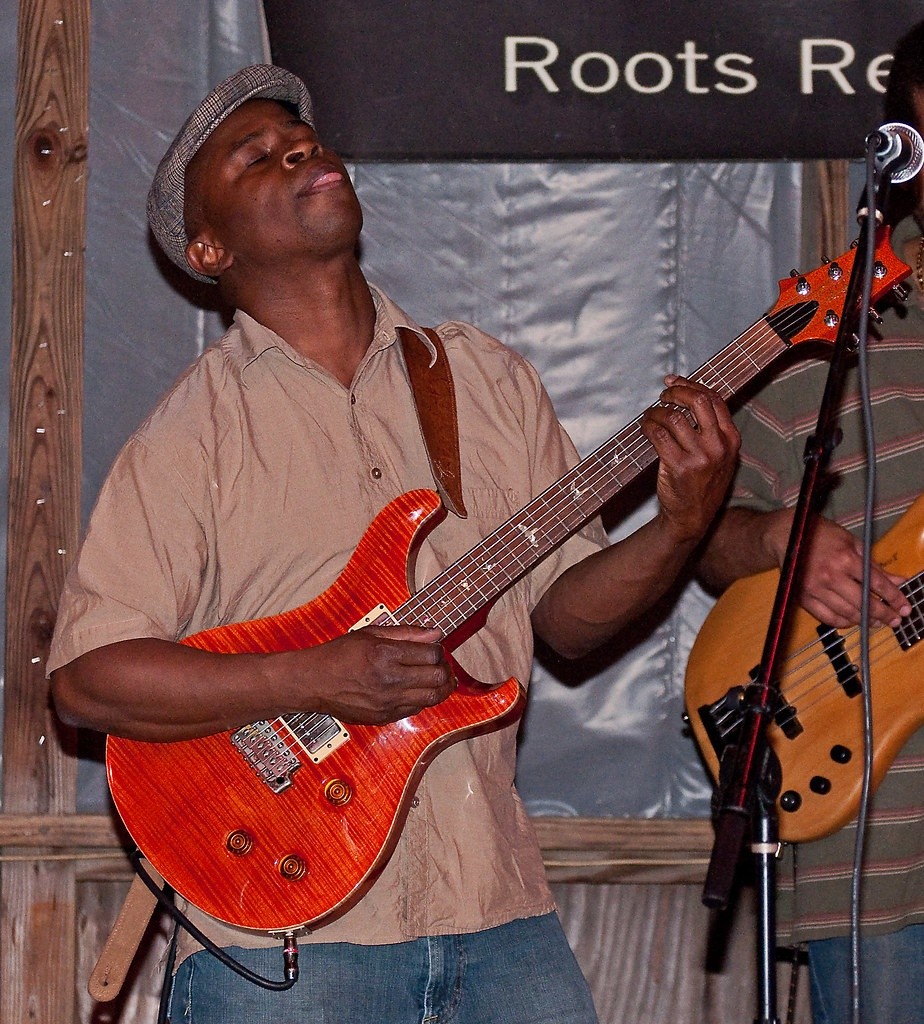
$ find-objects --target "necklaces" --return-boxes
[911,237,924,291]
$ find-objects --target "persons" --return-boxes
[689,21,924,1024]
[44,66,742,1024]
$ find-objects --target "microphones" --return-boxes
[865,123,924,183]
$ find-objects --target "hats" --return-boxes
[145,65,318,284]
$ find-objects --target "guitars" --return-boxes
[682,491,924,844]
[103,218,916,942]
